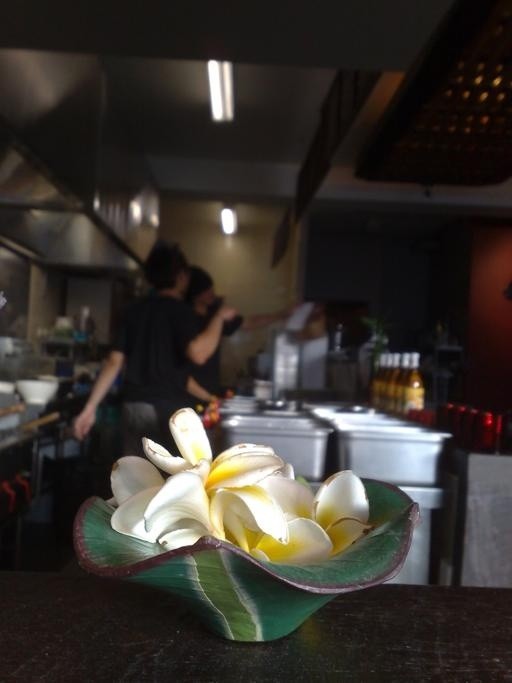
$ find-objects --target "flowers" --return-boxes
[109,403,373,565]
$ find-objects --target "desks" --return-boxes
[0,575,512,682]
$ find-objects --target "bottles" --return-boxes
[370,352,424,416]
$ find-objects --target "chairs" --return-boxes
[11,427,90,567]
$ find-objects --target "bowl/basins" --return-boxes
[15,379,58,408]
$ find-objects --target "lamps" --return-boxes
[207,53,236,125]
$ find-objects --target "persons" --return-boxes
[186,256,304,401]
[69,238,240,474]
[279,293,390,402]
